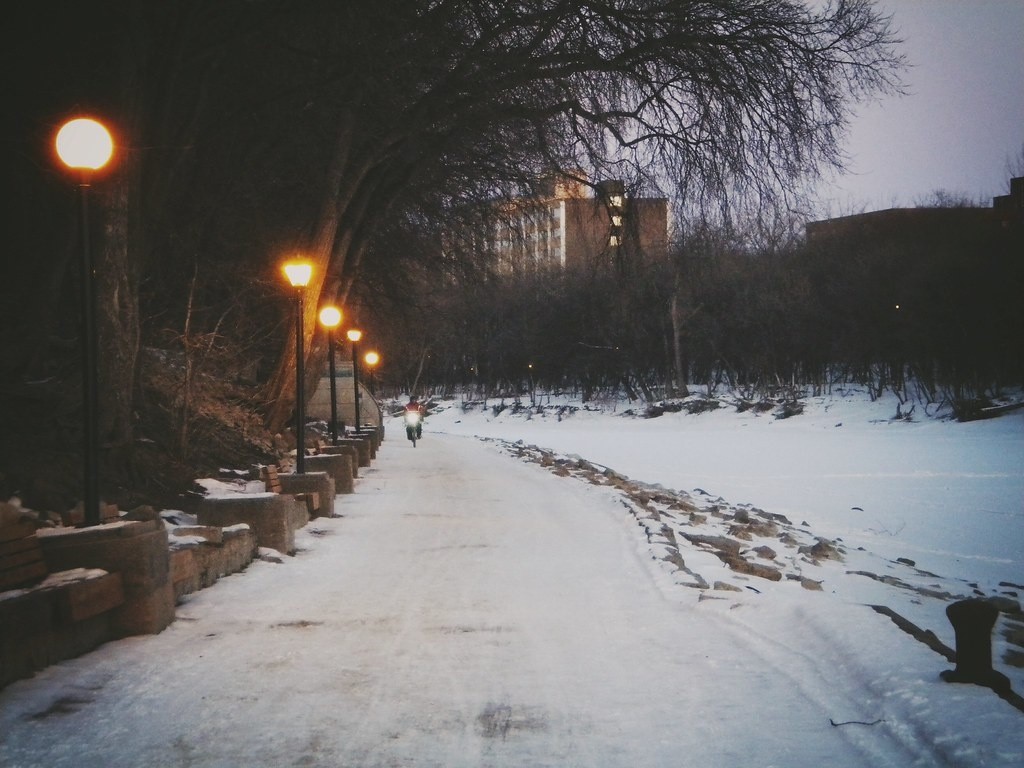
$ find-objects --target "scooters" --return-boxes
[403,405,422,447]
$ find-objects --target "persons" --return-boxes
[405,396,424,441]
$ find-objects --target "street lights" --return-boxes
[281,253,316,476]
[319,306,342,445]
[53,116,113,525]
[348,328,362,432]
[364,351,377,394]
[527,362,534,402]
